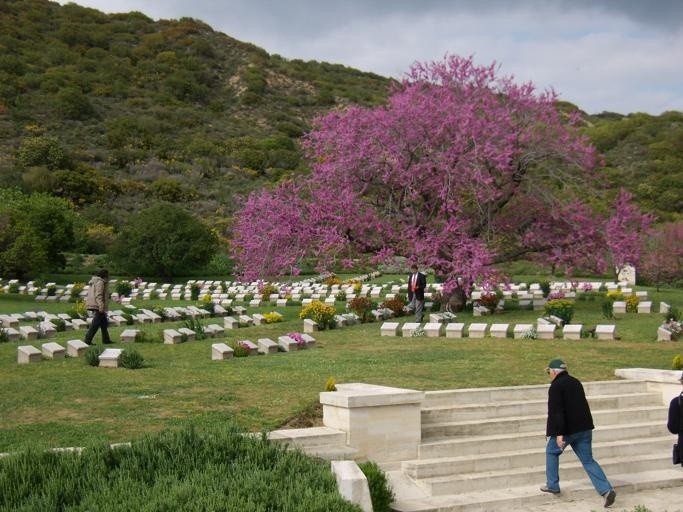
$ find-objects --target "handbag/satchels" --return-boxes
[673,444,681,464]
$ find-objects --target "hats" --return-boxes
[544,359,566,373]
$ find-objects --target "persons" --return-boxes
[82,269,117,346]
[666,371,683,470]
[406,264,426,323]
[538,359,615,508]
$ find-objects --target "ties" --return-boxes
[412,273,415,292]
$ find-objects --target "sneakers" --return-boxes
[540,485,560,493]
[85,342,97,346]
[102,341,117,344]
[603,490,616,507]
